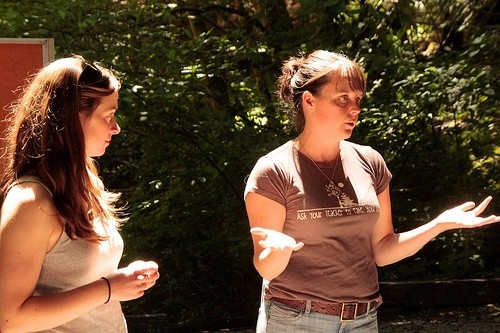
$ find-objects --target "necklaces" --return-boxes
[298,135,340,188]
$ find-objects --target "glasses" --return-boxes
[79,59,102,87]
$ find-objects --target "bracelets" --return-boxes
[100,276,111,304]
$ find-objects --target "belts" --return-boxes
[272,296,383,321]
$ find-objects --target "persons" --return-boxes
[0,51,162,333]
[242,49,499,333]
